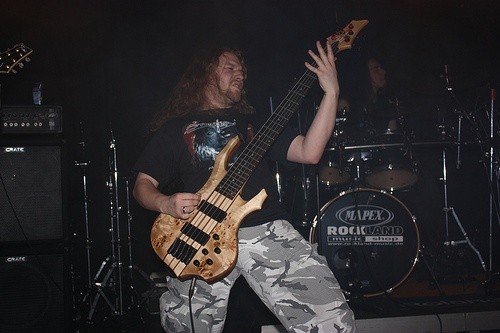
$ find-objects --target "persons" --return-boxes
[132,35,356,333]
[336,52,397,135]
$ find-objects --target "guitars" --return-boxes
[149,17,370,284]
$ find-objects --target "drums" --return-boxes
[363,129,421,191]
[304,134,358,193]
[309,187,420,299]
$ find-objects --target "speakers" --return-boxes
[0,136,74,333]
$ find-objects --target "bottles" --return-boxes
[32,74,48,105]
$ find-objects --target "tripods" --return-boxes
[81,124,157,330]
[438,144,487,273]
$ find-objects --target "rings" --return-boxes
[182,206,186,213]
